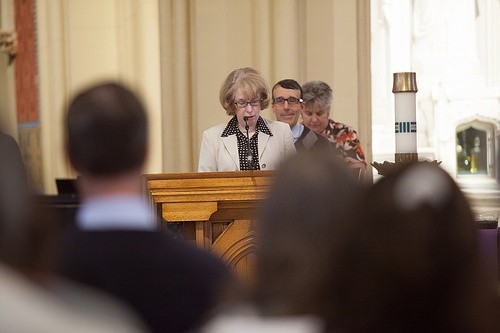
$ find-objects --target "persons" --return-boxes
[18,75,239,332]
[198,134,382,333]
[268,77,343,173]
[295,80,368,173]
[196,65,301,173]
[305,156,492,333]
[1,121,144,331]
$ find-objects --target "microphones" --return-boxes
[244,116,253,170]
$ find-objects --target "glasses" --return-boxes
[232,99,264,107]
[273,96,303,106]
